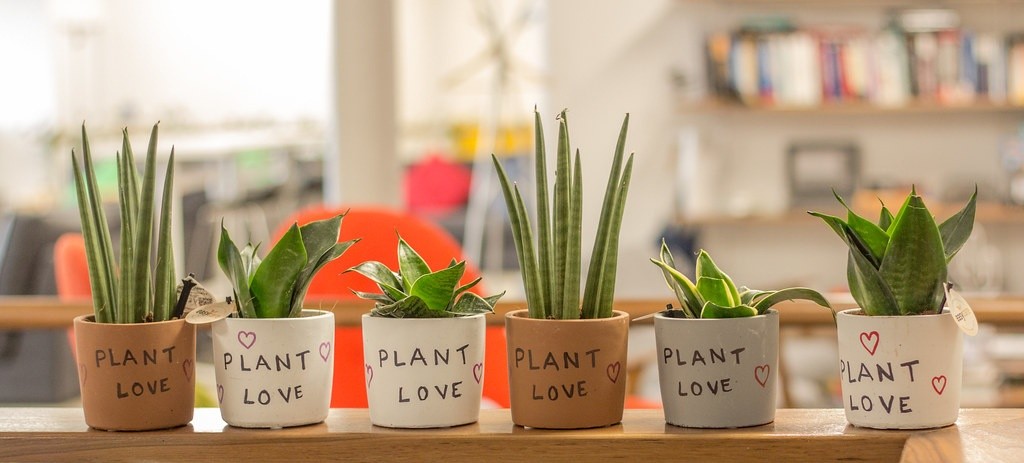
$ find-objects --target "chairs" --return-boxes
[267,206,659,411]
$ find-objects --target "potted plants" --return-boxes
[492,101,632,430]
[808,181,981,432]
[342,231,507,430]
[647,238,831,428]
[212,210,338,429]
[68,115,198,433]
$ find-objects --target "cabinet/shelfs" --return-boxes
[664,97,1024,227]
